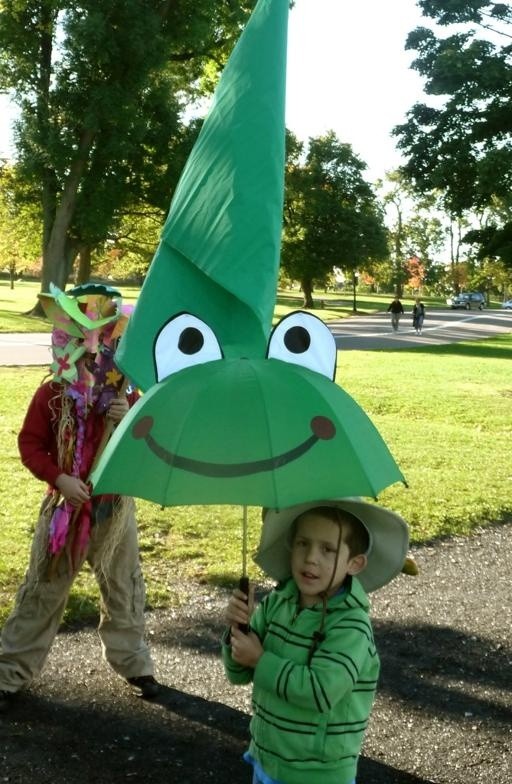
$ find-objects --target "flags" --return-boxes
[113,0,295,394]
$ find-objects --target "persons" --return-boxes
[385,295,404,330]
[3,282,169,704]
[411,298,426,336]
[219,498,409,784]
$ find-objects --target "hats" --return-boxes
[252,498,410,595]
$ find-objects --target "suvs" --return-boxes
[451,292,486,311]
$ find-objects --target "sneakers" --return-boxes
[117,672,160,698]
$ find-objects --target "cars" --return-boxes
[502,298,512,309]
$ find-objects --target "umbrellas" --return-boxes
[78,310,415,637]
